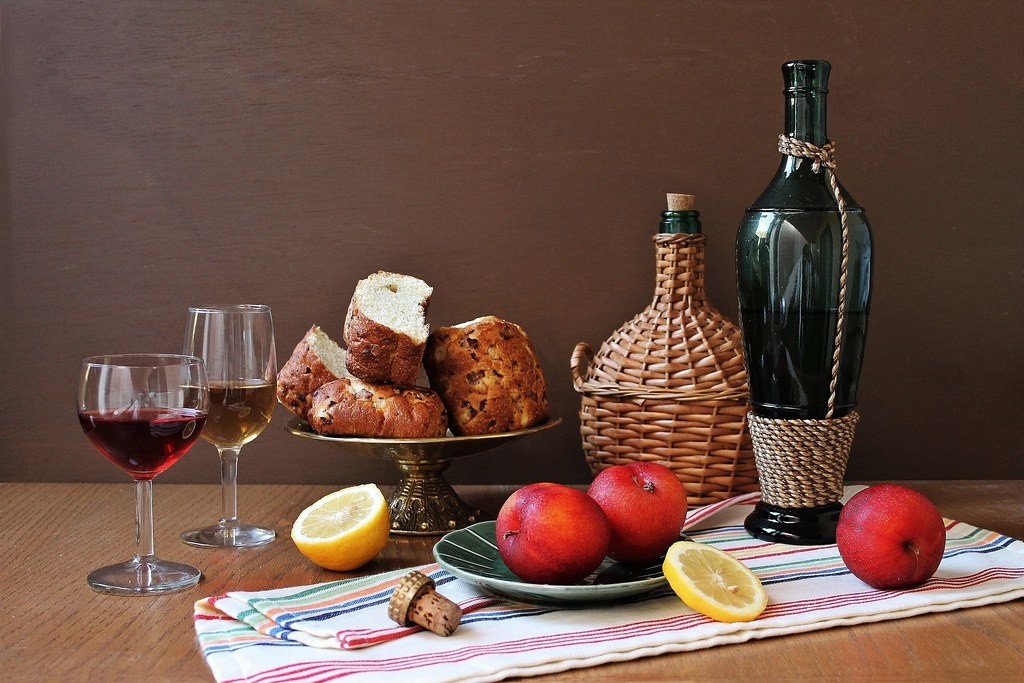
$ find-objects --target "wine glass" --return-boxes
[178,303,279,549]
[75,350,213,595]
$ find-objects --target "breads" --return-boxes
[277,325,352,421]
[424,315,547,433]
[344,269,435,384]
[308,380,447,438]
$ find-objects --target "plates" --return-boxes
[431,519,696,605]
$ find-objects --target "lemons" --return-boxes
[291,484,390,570]
[663,541,768,620]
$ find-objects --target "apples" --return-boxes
[496,484,611,584]
[588,463,687,559]
[837,484,945,589]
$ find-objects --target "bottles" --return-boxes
[733,57,878,547]
[657,192,702,234]
